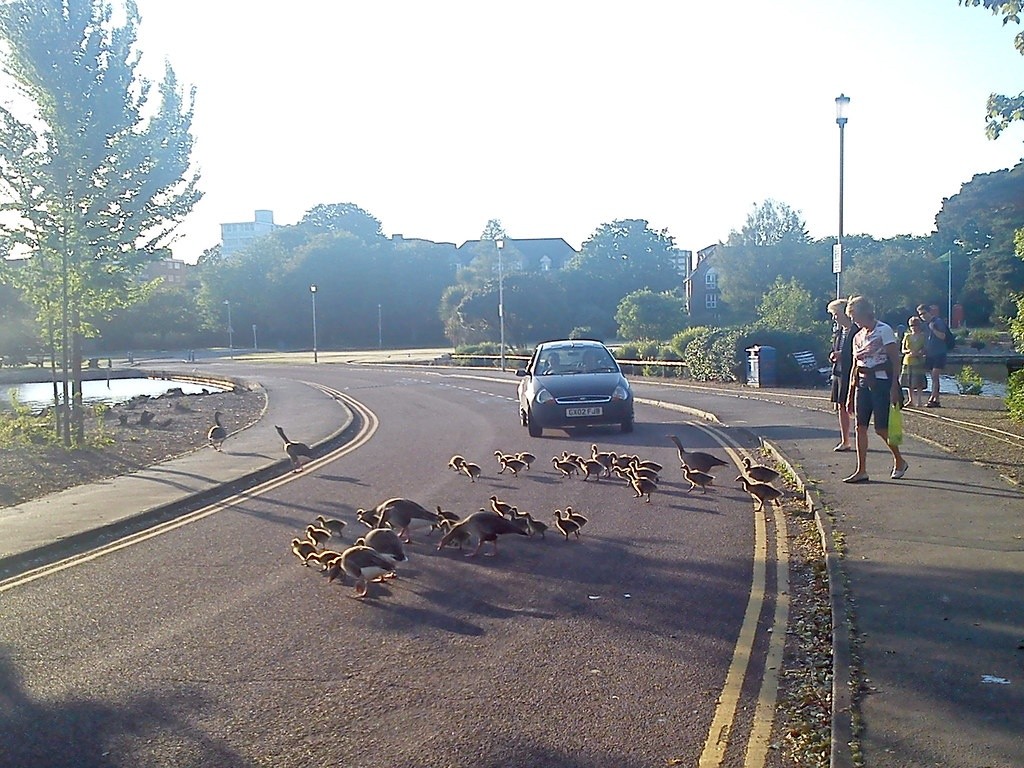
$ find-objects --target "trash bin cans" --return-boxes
[745,344,776,388]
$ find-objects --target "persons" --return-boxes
[583,350,593,367]
[842,297,908,483]
[952,301,963,328]
[542,352,567,371]
[827,299,859,452]
[916,304,947,407]
[899,317,927,407]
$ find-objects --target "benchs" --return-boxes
[786,351,833,386]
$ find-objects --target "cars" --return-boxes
[514,340,636,438]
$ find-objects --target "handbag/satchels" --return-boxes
[935,317,956,350]
[887,403,904,445]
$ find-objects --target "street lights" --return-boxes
[495,238,507,372]
[310,284,317,362]
[833,93,851,298]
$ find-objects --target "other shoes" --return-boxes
[843,475,868,483]
[925,401,941,408]
[904,401,916,407]
[891,460,908,478]
[916,400,924,407]
[834,444,850,452]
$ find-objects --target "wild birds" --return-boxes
[207,411,227,452]
[733,457,784,513]
[679,464,717,496]
[553,510,581,543]
[565,507,588,529]
[665,435,731,487]
[290,515,407,599]
[357,498,472,550]
[448,455,482,483]
[479,495,549,540]
[275,425,315,473]
[550,444,663,503]
[437,511,530,558]
[494,450,536,477]
